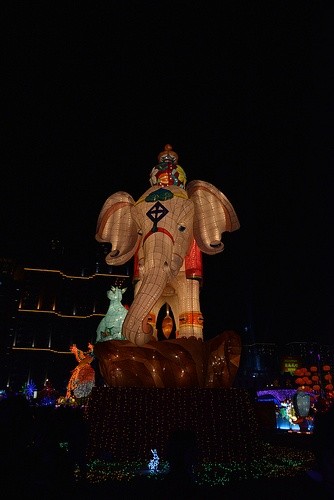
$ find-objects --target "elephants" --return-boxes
[95,180,241,343]
